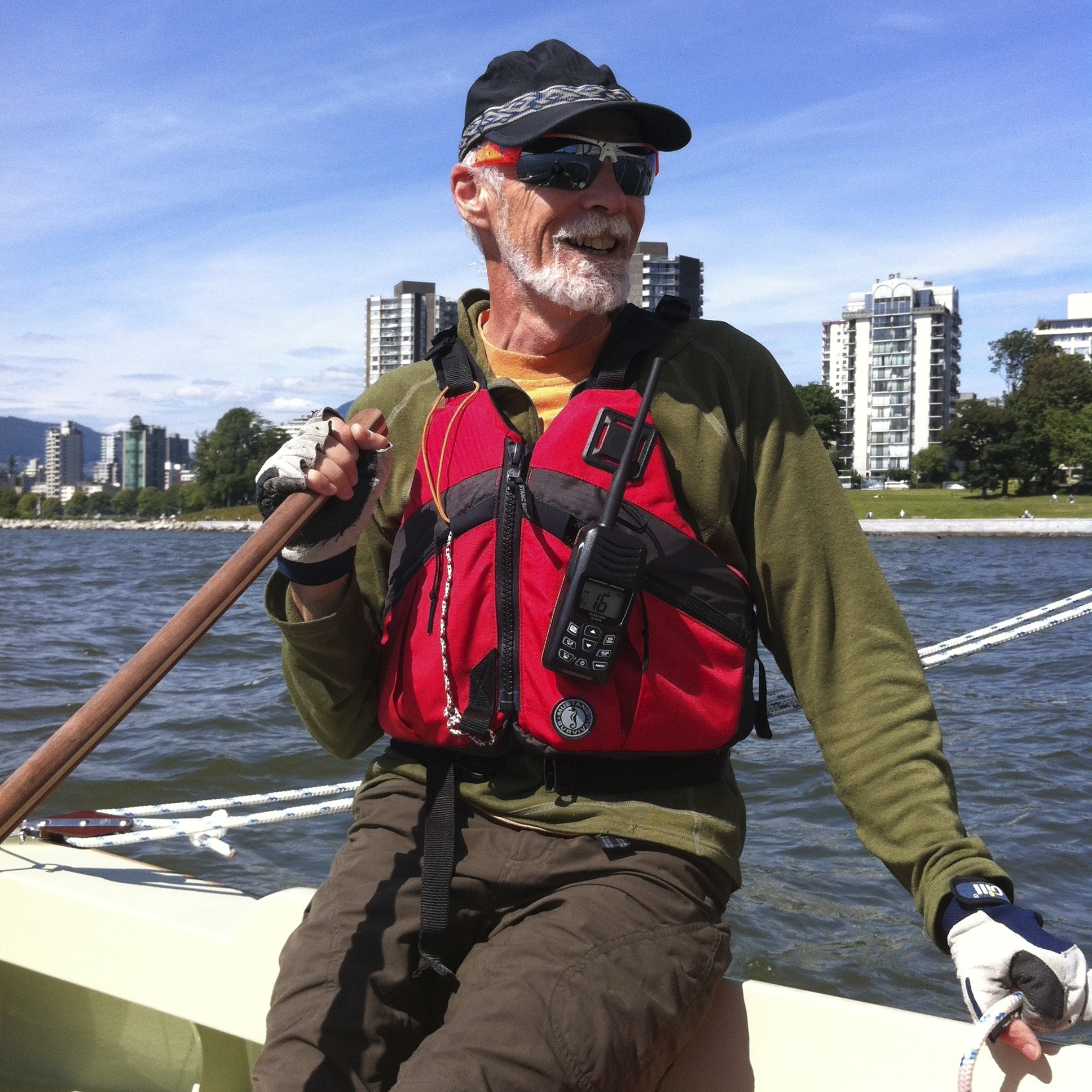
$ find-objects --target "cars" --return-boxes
[947,484,965,490]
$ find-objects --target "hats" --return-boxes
[456,37,693,164]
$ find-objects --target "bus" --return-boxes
[838,475,855,490]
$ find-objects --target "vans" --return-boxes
[883,481,910,490]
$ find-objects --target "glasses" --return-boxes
[470,132,660,196]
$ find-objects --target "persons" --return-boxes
[866,511,873,519]
[1020,509,1033,518]
[900,509,905,518]
[169,513,176,522]
[873,494,883,498]
[1050,493,1058,502]
[1069,492,1075,504]
[252,38,1092,1092]
[160,513,167,522]
